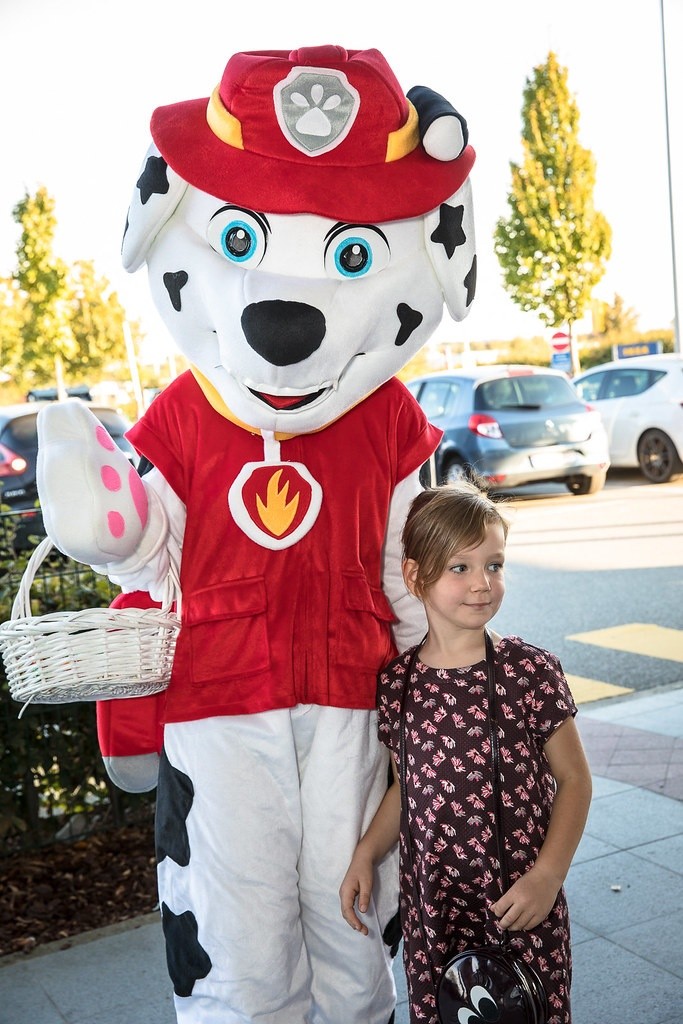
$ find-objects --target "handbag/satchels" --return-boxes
[435,945,548,1024]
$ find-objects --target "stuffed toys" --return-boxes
[35,46,479,1024]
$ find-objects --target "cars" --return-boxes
[544,353,683,484]
[397,365,611,499]
[0,400,139,517]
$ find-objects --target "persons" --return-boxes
[338,485,592,1024]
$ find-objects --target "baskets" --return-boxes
[0,536,181,703]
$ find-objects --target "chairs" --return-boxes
[615,376,638,396]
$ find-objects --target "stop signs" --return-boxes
[550,331,571,351]
